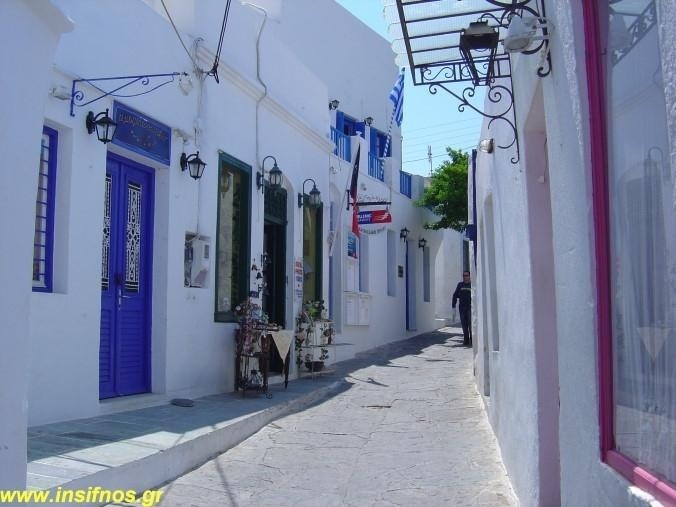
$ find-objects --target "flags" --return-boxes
[350,142,361,238]
[387,72,402,127]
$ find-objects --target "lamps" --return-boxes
[298,177,323,212]
[399,226,409,242]
[478,137,497,153]
[255,153,285,194]
[87,105,117,145]
[456,12,554,89]
[419,236,427,251]
[180,149,206,181]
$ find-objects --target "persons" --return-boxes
[452,270,471,346]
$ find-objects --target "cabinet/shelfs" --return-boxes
[234,317,273,396]
[295,312,334,384]
